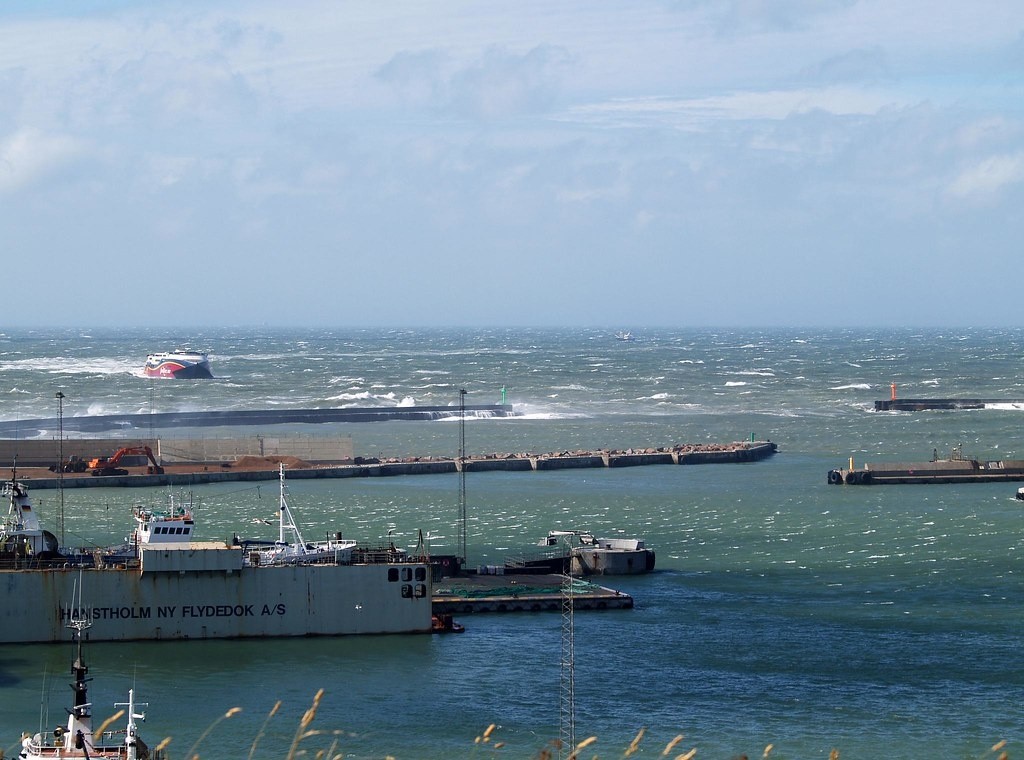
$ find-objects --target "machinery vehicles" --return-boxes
[48,454,87,474]
[84,446,165,476]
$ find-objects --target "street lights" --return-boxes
[458,389,469,570]
[55,391,66,548]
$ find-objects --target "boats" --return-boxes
[0,453,357,563]
[432,612,466,634]
[0,564,150,760]
[144,348,213,379]
